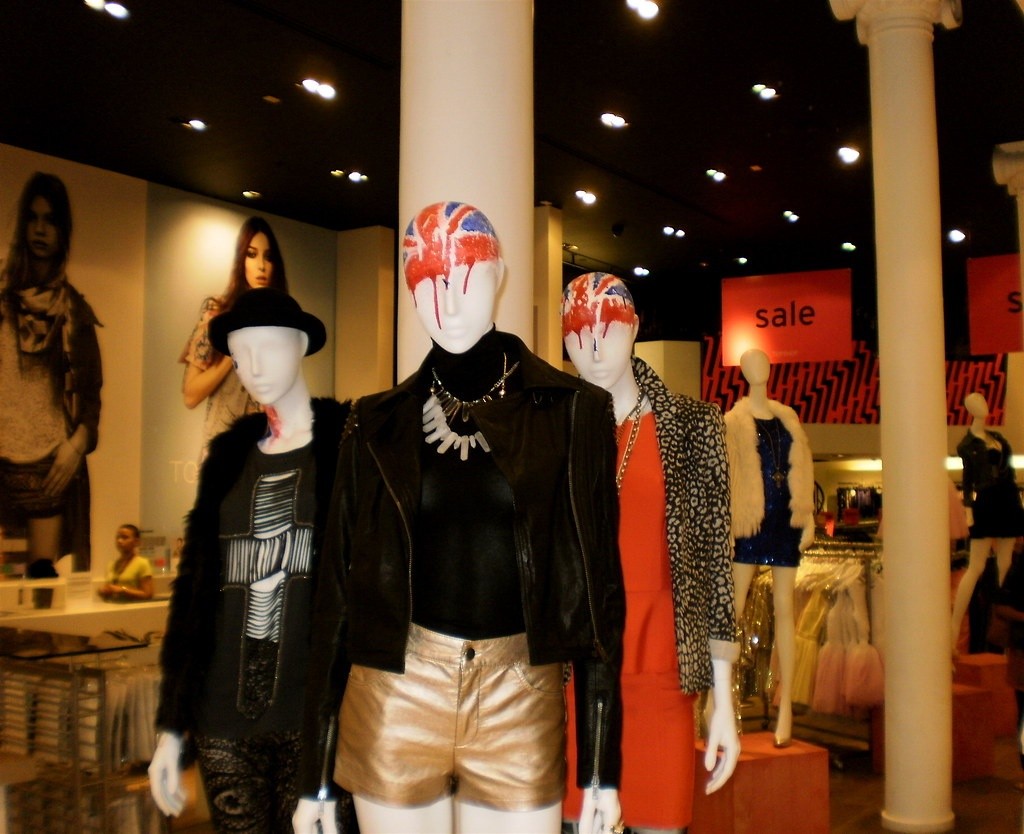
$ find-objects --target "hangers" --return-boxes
[764,540,883,589]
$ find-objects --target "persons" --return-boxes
[0,171,104,576]
[292,201,627,834]
[97,525,153,604]
[173,538,183,559]
[559,271,742,834]
[950,393,1024,650]
[147,286,351,834]
[178,216,288,461]
[704,348,813,750]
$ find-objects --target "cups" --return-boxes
[154,546,170,575]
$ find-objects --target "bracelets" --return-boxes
[120,586,127,596]
[68,440,82,455]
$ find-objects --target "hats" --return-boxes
[207,287,327,357]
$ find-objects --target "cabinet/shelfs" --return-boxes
[0,637,150,834]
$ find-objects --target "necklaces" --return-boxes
[753,415,784,488]
[616,376,643,496]
[428,354,519,423]
[987,432,1000,449]
